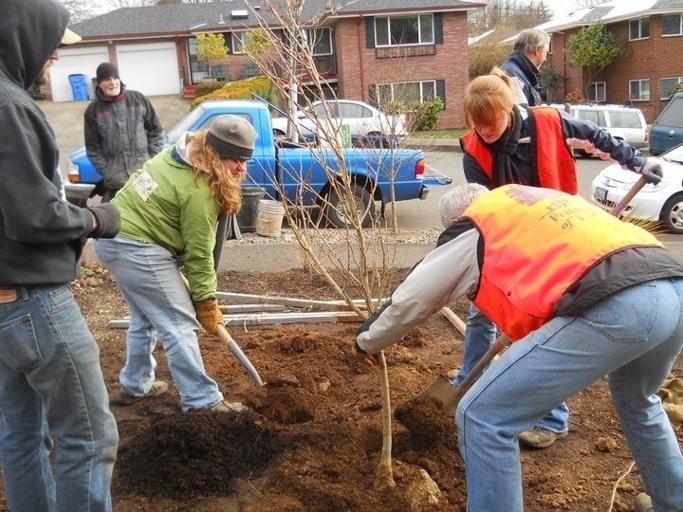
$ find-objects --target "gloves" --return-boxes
[85,203,122,238]
[641,159,664,185]
[192,298,226,336]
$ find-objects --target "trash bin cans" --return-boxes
[69,74,90,101]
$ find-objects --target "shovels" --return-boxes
[395,167,656,442]
[180,272,318,424]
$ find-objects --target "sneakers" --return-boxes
[207,400,248,415]
[635,492,655,512]
[116,381,169,404]
[518,424,568,448]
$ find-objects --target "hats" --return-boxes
[59,27,83,46]
[207,114,258,160]
[96,62,120,88]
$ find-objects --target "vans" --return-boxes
[541,103,650,157]
[648,92,683,154]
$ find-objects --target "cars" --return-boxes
[591,143,683,234]
[272,99,409,149]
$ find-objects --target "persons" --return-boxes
[83,62,164,203]
[91,114,252,415]
[497,27,551,106]
[354,183,682,511]
[451,66,661,449]
[0,1,119,511]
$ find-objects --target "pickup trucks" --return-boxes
[62,99,453,230]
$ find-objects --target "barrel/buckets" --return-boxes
[255,202,285,238]
[236,187,266,232]
